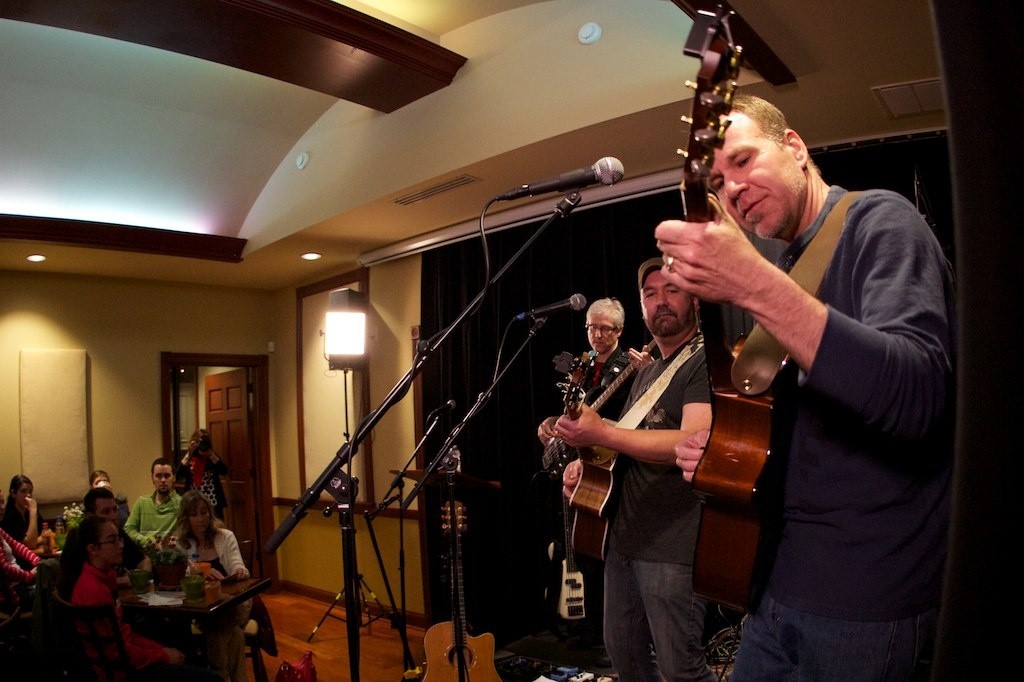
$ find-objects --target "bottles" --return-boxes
[186,554,200,564]
[42,523,53,554]
[55,515,64,536]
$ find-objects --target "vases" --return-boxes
[153,563,188,591]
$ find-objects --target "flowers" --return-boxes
[142,532,190,569]
[55,502,85,550]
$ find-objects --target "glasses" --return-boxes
[93,538,124,549]
[92,505,120,518]
[585,320,618,338]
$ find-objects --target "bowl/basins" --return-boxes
[191,562,210,579]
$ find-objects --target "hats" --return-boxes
[638,257,662,289]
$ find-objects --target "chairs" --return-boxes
[0,540,269,682]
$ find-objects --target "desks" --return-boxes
[117,577,272,682]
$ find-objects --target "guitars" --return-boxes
[421,444,503,682]
[556,350,620,561]
[676,2,776,614]
[557,490,586,620]
[541,335,659,471]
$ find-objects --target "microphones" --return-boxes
[517,293,585,320]
[426,398,456,418]
[506,155,623,198]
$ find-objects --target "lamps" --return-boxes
[319,288,368,370]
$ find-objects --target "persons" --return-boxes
[0,429,250,682]
[537,297,654,667]
[655,94,956,682]
[555,257,718,682]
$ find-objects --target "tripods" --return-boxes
[306,574,388,642]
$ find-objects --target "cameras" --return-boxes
[193,435,214,452]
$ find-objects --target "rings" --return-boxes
[666,257,673,271]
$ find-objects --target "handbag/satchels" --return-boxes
[273,650,317,682]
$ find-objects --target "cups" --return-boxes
[204,579,221,602]
[128,569,150,594]
[54,536,67,550]
[180,572,205,603]
